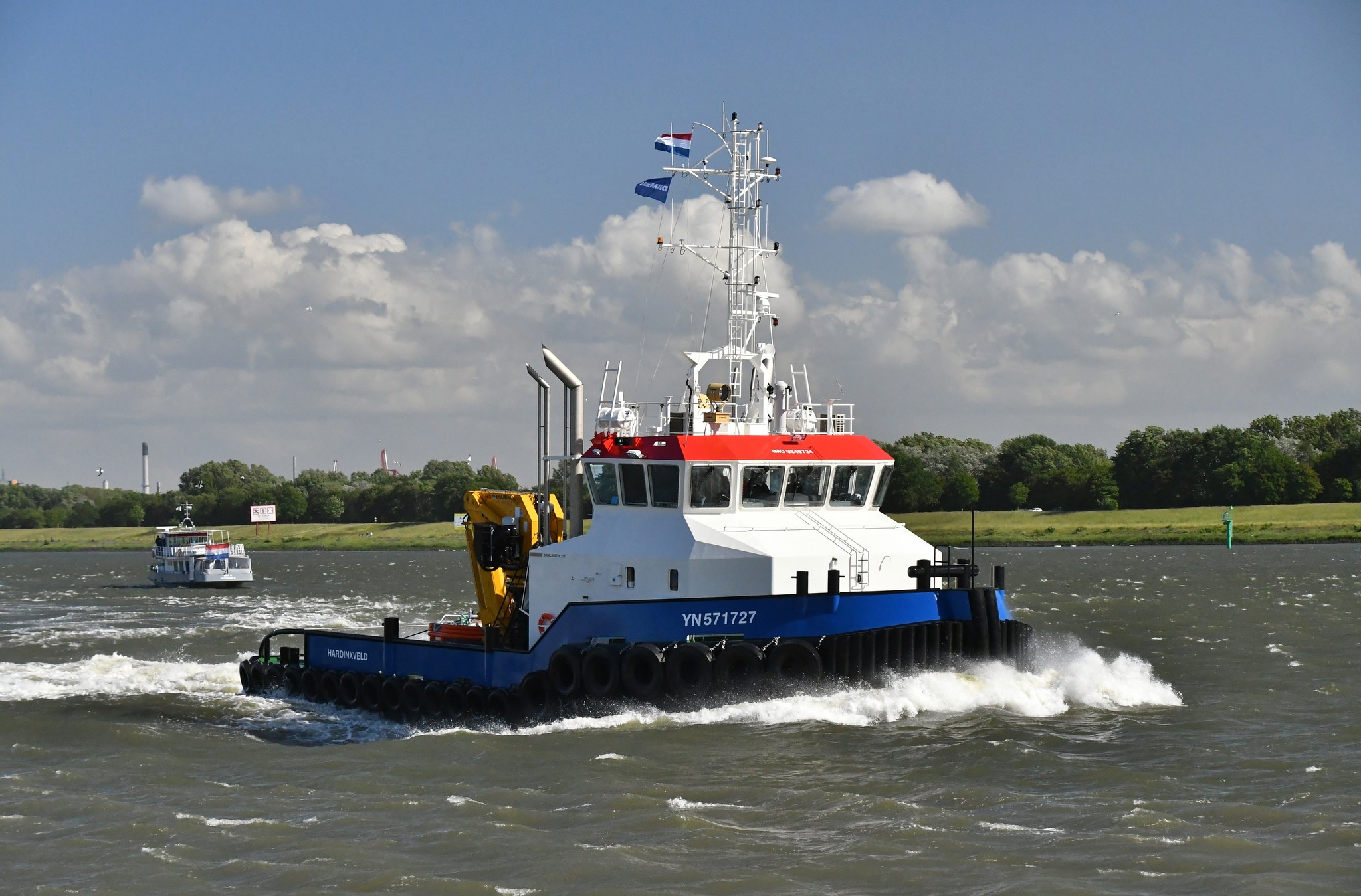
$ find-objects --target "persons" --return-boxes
[155,536,162,546]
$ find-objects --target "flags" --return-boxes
[635,176,671,204]
[206,543,229,560]
[655,132,692,159]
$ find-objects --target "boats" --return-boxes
[234,103,1036,725]
[147,499,256,588]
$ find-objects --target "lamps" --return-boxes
[790,432,808,444]
[570,576,584,582]
[759,156,777,164]
[753,275,761,284]
[774,167,780,176]
[587,576,595,583]
[581,575,589,581]
[757,122,764,131]
[722,270,729,284]
[592,448,601,456]
[772,317,780,327]
[756,199,762,207]
[626,449,646,460]
[773,242,780,250]
[724,194,731,203]
[657,237,663,244]
[730,112,738,121]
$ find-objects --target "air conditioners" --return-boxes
[815,413,845,435]
